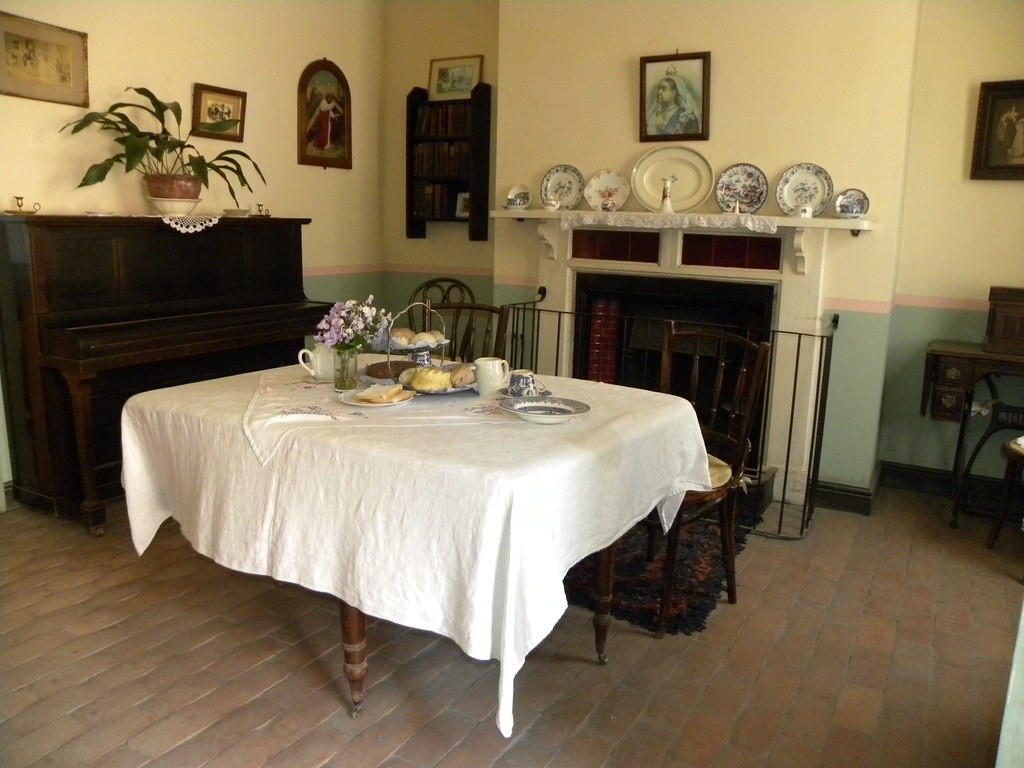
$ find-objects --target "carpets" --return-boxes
[563,499,765,636]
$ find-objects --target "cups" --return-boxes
[413,351,430,367]
[794,206,812,217]
[508,198,528,207]
[508,370,546,397]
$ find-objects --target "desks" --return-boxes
[117,354,732,739]
[918,338,1024,530]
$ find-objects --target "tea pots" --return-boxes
[542,197,561,211]
[297,344,335,383]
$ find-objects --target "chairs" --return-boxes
[423,297,511,364]
[408,277,477,363]
[589,318,772,667]
[983,434,1024,549]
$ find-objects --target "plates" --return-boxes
[503,205,528,210]
[714,163,768,214]
[397,362,478,395]
[508,184,532,207]
[831,213,868,219]
[499,397,591,424]
[583,169,631,212]
[540,165,583,210]
[339,390,414,407]
[498,388,553,398]
[631,146,714,213]
[776,163,834,217]
[836,189,869,214]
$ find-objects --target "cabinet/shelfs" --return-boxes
[404,82,492,241]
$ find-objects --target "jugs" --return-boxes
[474,357,509,394]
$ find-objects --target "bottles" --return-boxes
[601,192,617,211]
[659,178,675,213]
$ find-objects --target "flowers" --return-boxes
[312,293,394,390]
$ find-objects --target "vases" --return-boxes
[331,345,363,394]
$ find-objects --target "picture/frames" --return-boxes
[297,57,353,170]
[425,54,483,102]
[970,79,1024,181]
[455,192,470,218]
[190,83,247,143]
[638,48,711,144]
[0,9,90,109]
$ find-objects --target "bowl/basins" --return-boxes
[223,209,249,216]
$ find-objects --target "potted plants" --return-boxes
[57,86,269,209]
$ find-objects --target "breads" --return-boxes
[390,328,445,347]
[366,361,423,379]
[354,384,417,403]
[399,365,475,391]
